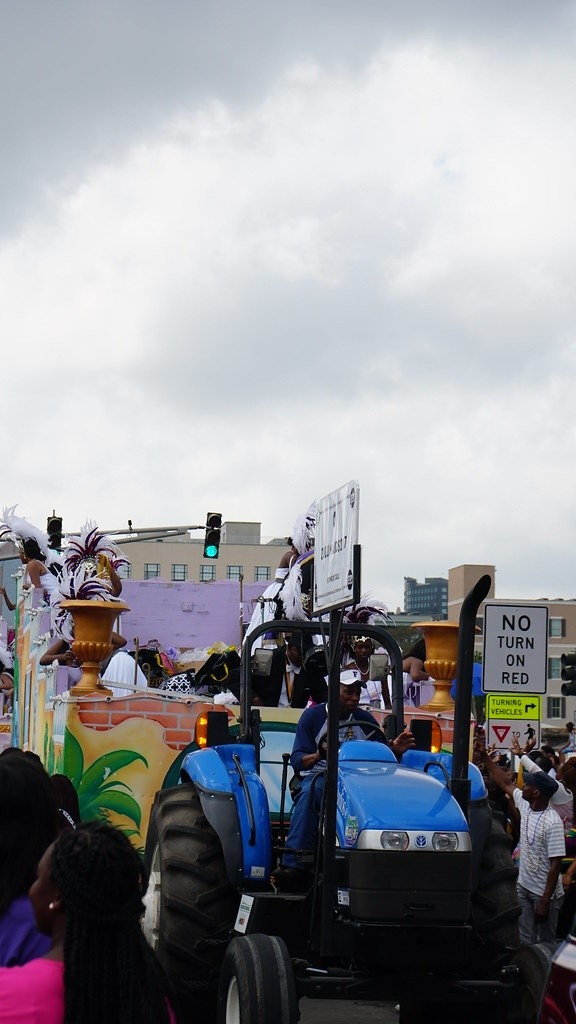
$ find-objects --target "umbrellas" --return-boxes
[451,662,486,698]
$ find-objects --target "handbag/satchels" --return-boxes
[187,646,240,704]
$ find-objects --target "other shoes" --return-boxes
[280,865,311,892]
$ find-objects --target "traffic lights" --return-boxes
[560,651,576,697]
[47,515,63,555]
[202,512,223,559]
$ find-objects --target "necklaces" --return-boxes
[525,804,552,873]
[353,660,371,674]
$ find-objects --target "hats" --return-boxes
[285,631,316,649]
[324,649,368,689]
[341,594,398,651]
[289,498,318,554]
[1,507,133,606]
[523,771,558,798]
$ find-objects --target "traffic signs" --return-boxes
[486,693,543,720]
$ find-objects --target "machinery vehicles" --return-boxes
[10,545,564,1024]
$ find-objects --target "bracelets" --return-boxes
[519,753,524,758]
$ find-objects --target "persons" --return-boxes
[558,722,576,770]
[0,660,14,690]
[0,748,81,966]
[270,669,416,885]
[242,549,329,709]
[339,633,393,710]
[0,587,17,611]
[473,744,566,945]
[473,735,576,890]
[0,823,175,1024]
[18,536,128,668]
[392,639,429,706]
